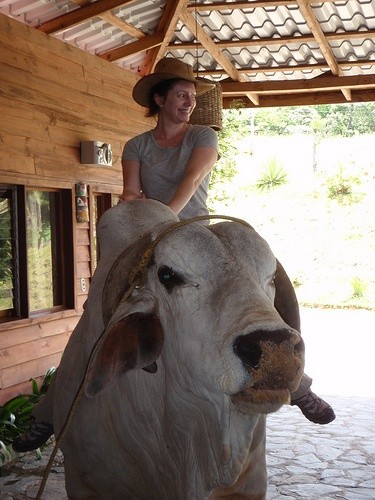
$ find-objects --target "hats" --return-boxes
[132,57,216,107]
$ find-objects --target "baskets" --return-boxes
[188,76,224,131]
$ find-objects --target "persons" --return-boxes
[12,56,337,455]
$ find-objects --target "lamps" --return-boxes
[185,1,223,129]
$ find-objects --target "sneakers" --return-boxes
[12,420,54,453]
[290,390,336,425]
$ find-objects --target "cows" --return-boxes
[57,197,304,500]
